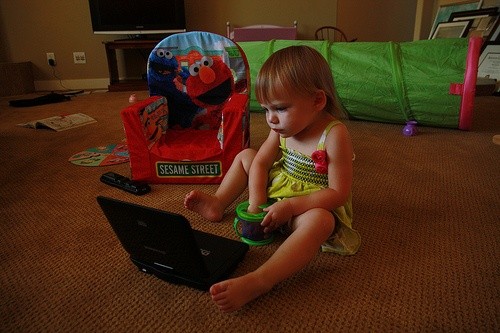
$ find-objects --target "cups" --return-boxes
[233,202,274,246]
[402,121,418,136]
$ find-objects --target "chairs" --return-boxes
[315,26,357,41]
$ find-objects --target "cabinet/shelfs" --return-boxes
[104,37,163,92]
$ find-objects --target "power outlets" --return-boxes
[47,53,55,64]
[73,52,86,64]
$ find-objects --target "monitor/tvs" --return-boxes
[88,0,186,41]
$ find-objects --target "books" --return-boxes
[16,112,98,132]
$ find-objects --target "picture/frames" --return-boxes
[427,0,500,81]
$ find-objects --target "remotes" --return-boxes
[100,172,152,195]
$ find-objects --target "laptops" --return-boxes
[97,195,250,289]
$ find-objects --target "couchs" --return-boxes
[120,30,249,184]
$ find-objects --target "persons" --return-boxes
[184,44,361,313]
